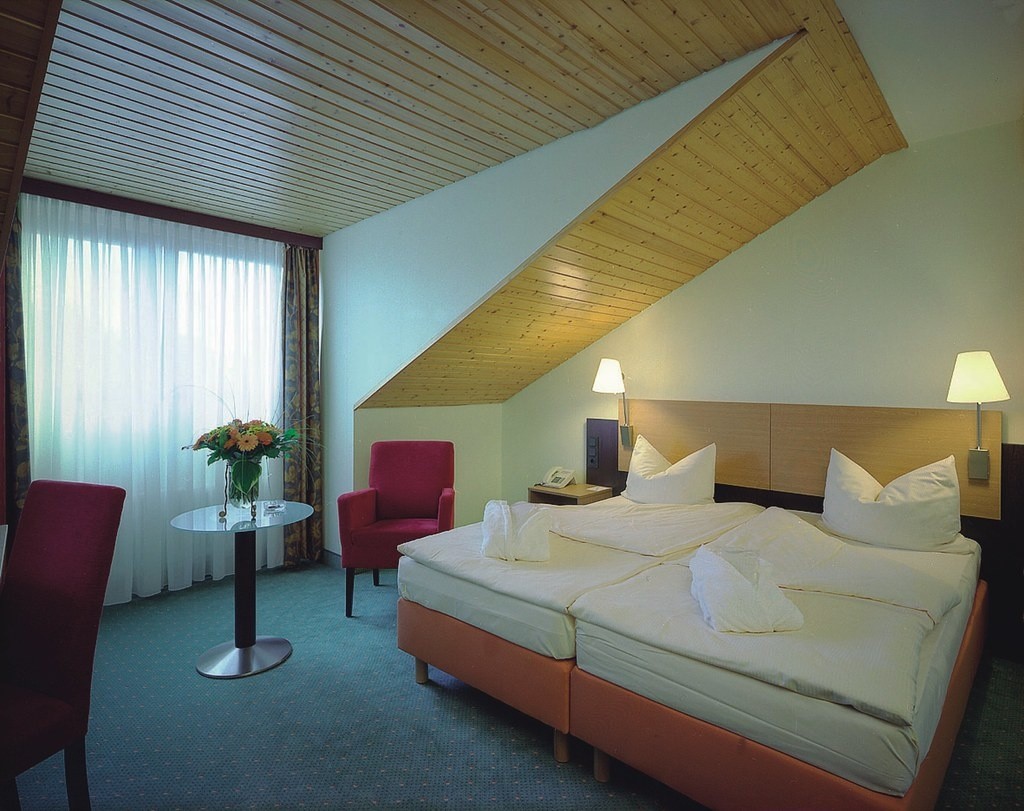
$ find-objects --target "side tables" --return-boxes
[528,483,612,505]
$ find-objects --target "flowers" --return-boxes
[180,378,326,518]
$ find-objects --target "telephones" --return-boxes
[543,467,576,488]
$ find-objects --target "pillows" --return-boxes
[816,447,976,556]
[620,433,716,505]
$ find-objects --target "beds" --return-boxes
[397,398,1002,810]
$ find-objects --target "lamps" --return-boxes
[946,350,1010,480]
[591,358,634,447]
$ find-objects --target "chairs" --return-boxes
[0,480,127,811]
[337,440,455,618]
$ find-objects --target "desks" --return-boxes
[170,500,314,679]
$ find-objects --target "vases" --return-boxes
[229,467,258,509]
